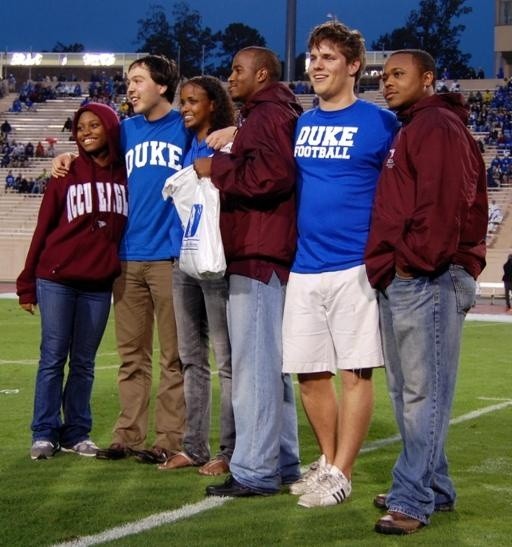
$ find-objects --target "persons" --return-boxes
[16,102,129,461]
[435,66,512,233]
[50,56,239,465]
[288,79,315,94]
[0,70,136,194]
[366,47,488,534]
[501,254,512,311]
[281,21,404,508]
[157,74,236,477]
[192,45,304,498]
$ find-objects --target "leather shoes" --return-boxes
[374,512,422,534]
[376,494,455,511]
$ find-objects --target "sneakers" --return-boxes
[30,438,228,476]
[207,456,351,509]
